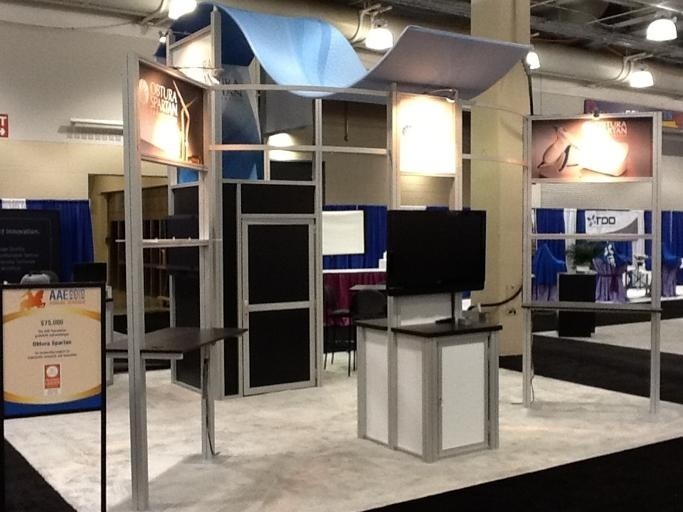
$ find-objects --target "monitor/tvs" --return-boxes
[0,209,63,282]
[386,209,487,297]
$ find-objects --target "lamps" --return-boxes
[364,17,393,53]
[645,17,677,42]
[628,60,653,89]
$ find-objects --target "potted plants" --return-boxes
[564,241,610,273]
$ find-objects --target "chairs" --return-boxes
[323,284,356,377]
